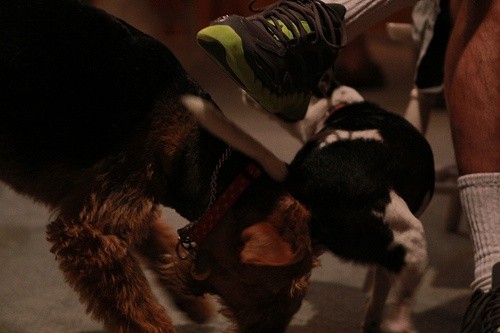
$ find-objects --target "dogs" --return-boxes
[0,0,436,333]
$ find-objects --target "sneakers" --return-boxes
[331,60,386,89]
[198,2,346,125]
[463,263,500,333]
